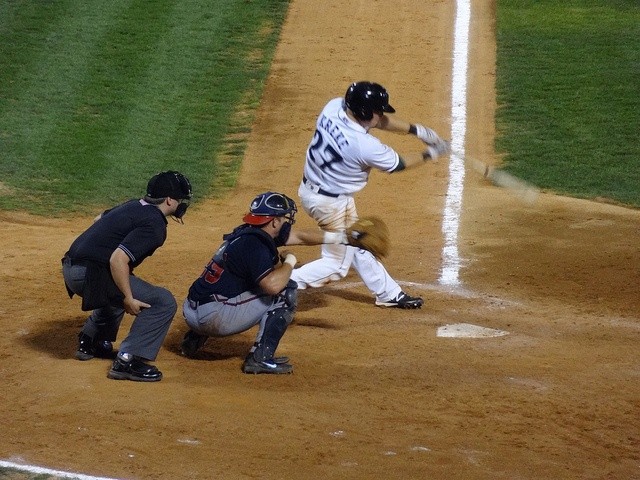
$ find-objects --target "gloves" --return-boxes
[417,123,445,147]
[427,141,449,162]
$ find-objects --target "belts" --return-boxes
[303,174,339,198]
[62,259,79,266]
[188,295,215,306]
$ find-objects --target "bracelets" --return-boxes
[408,123,417,135]
[422,149,431,161]
[284,254,296,268]
[323,231,342,244]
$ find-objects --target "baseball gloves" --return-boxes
[344,215,392,260]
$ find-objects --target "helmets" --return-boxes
[345,81,396,121]
[146,170,194,225]
[242,192,298,247]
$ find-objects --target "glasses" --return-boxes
[284,216,296,225]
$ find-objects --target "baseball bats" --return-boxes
[449,146,541,207]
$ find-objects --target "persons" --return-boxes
[182,192,359,373]
[288,82,438,309]
[62,170,193,382]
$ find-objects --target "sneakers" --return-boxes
[375,289,424,309]
[75,341,120,360]
[241,348,293,374]
[179,339,197,358]
[108,355,163,381]
